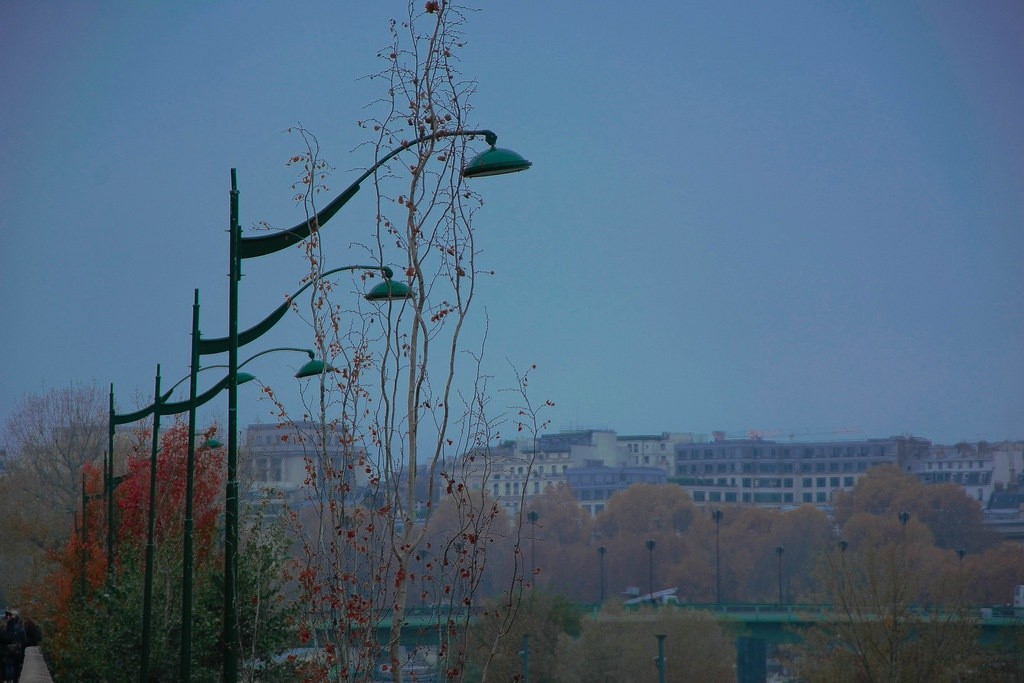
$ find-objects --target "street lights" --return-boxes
[526,510,539,592]
[81,429,223,602]
[645,536,657,603]
[899,507,911,572]
[105,368,255,618]
[596,545,606,606]
[774,545,787,611]
[956,546,966,611]
[221,128,534,683]
[178,266,421,683]
[711,507,723,604]
[418,549,429,615]
[454,540,466,608]
[142,343,342,683]
[837,537,850,611]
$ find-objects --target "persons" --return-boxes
[0,618,22,683]
[20,617,41,668]
[13,615,28,646]
[2,611,12,627]
